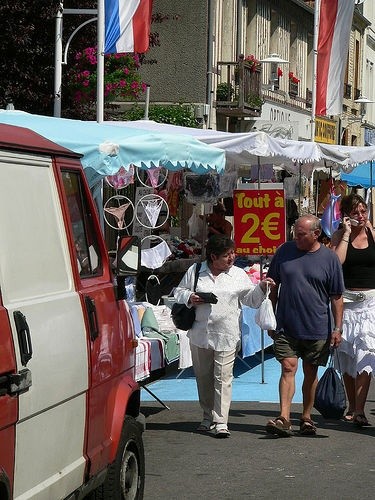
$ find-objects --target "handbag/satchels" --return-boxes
[169,263,202,331]
[312,343,347,421]
[254,279,278,331]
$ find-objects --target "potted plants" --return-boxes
[217,82,234,107]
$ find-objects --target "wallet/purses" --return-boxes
[194,292,218,305]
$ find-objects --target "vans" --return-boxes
[0,120,147,500]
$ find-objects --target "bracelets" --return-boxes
[333,327,343,334]
[341,235,349,243]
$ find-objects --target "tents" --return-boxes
[0,107,375,192]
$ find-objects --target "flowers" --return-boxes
[245,55,261,74]
[278,68,283,77]
[288,74,300,86]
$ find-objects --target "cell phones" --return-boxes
[344,213,359,226]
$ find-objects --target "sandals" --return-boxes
[298,417,316,435]
[266,416,293,438]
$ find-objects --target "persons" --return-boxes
[330,194,375,426]
[265,214,346,436]
[174,235,275,437]
[207,204,232,239]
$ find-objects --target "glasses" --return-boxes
[349,210,368,217]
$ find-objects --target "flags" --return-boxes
[315,0,355,115]
[104,0,153,55]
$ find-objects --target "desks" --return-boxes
[139,257,200,275]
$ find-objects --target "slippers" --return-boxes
[214,424,231,437]
[197,418,216,431]
[344,411,370,425]
